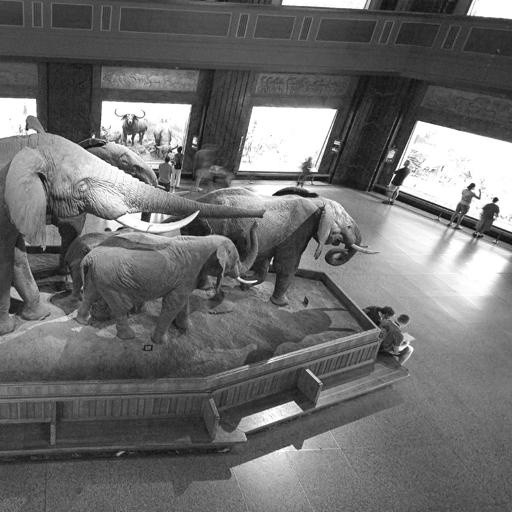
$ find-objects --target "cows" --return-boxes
[154,127,178,160]
[115,109,147,146]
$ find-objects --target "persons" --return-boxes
[157,156,173,192]
[446,182,482,230]
[361,304,395,326]
[297,156,315,188]
[377,314,414,367]
[471,196,500,238]
[171,145,185,190]
[380,159,411,206]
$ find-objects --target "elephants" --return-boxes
[209,186,380,309]
[2,130,205,346]
